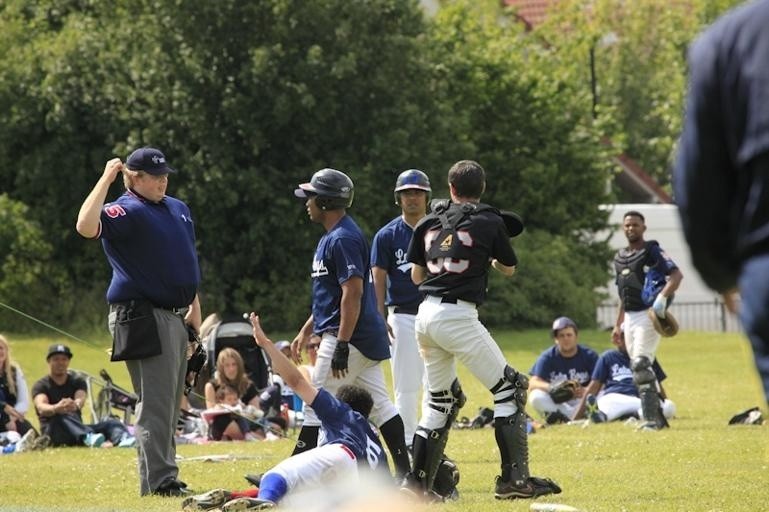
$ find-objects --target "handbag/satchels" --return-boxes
[111,300,162,361]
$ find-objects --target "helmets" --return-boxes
[552,316,577,338]
[394,169,432,192]
[641,270,674,310]
[298,168,354,199]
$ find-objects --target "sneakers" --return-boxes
[395,471,442,505]
[585,394,606,423]
[244,473,263,488]
[182,488,279,512]
[118,436,136,447]
[155,476,196,497]
[83,432,106,447]
[494,476,562,499]
[15,428,51,452]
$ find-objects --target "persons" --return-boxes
[1,335,135,453]
[368,167,443,481]
[727,406,769,430]
[611,212,684,431]
[181,310,393,511]
[398,158,561,506]
[526,315,674,434]
[244,166,413,490]
[676,1,769,408]
[174,312,322,445]
[76,145,203,498]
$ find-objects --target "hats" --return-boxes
[126,148,175,176]
[294,189,318,198]
[46,344,73,362]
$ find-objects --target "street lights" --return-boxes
[588,32,619,115]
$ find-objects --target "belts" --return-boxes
[394,307,418,315]
[109,301,178,312]
[441,297,457,303]
